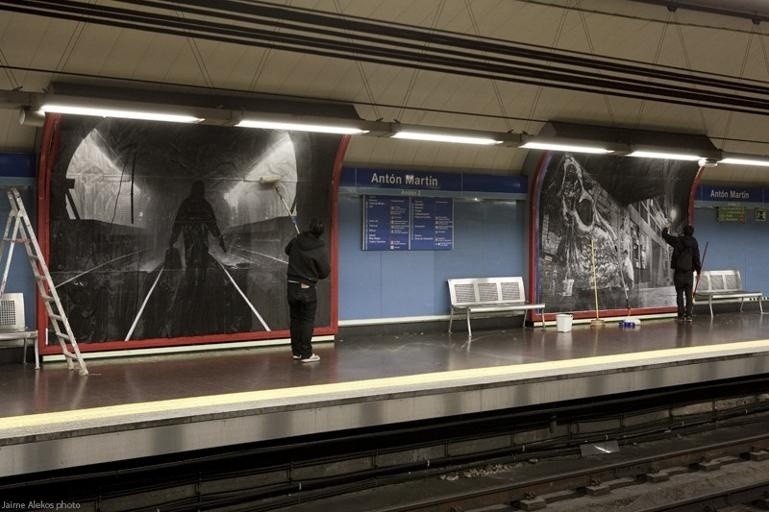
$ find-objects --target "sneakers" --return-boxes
[292,352,321,363]
[678,315,693,322]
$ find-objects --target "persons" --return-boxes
[662,222,701,322]
[169,177,226,284]
[284,218,331,363]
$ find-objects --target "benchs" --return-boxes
[0,293,40,370]
[693,270,764,321]
[447,276,547,339]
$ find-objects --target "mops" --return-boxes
[589,238,605,327]
[682,240,710,322]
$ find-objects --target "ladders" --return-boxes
[0,187,91,378]
